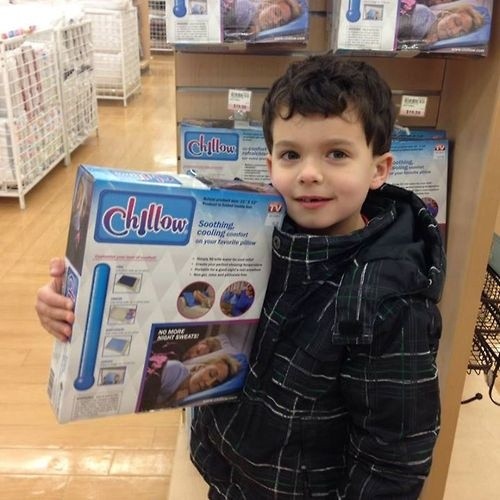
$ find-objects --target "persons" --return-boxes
[141,355,240,408]
[70,175,86,251]
[412,1,482,45]
[222,285,254,315]
[153,336,222,364]
[223,0,302,41]
[36,48,445,500]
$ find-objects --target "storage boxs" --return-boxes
[324,0,492,56]
[177,118,275,186]
[165,0,310,45]
[46,162,287,424]
[0,1,175,212]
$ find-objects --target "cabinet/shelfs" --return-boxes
[458,260,500,406]
